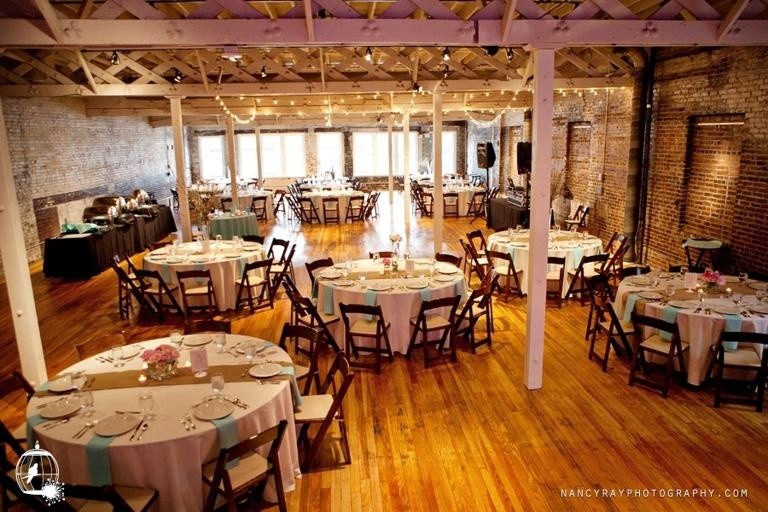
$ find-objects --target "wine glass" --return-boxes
[649,266,766,307]
[507,225,589,249]
[341,253,436,289]
[71,329,255,421]
[165,234,243,264]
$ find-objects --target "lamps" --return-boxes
[111,50,119,65]
[413,82,422,93]
[261,65,267,77]
[174,71,181,82]
[365,46,372,61]
[444,47,450,60]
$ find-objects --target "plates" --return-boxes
[321,257,458,292]
[151,240,259,263]
[630,275,768,315]
[40,333,284,437]
[497,228,598,249]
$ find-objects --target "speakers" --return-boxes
[516,140,532,175]
[476,141,496,169]
